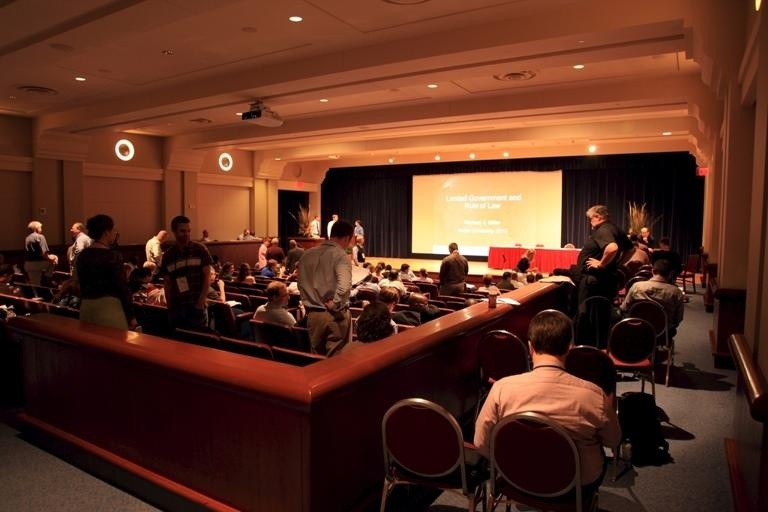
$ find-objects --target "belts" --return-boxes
[310,307,325,312]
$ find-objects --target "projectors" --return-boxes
[241,104,284,128]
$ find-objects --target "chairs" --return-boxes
[564,243,575,248]
[676,253,700,295]
[535,243,544,247]
[514,243,521,247]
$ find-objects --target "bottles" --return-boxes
[488,281,498,309]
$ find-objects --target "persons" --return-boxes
[353,217,365,259]
[66,223,96,277]
[296,220,354,359]
[614,228,682,339]
[25,221,56,265]
[577,204,636,315]
[349,243,469,343]
[327,214,339,239]
[80,214,138,331]
[475,248,578,296]
[155,216,214,330]
[122,229,168,304]
[472,310,623,504]
[310,216,321,239]
[10,264,79,307]
[199,229,304,326]
